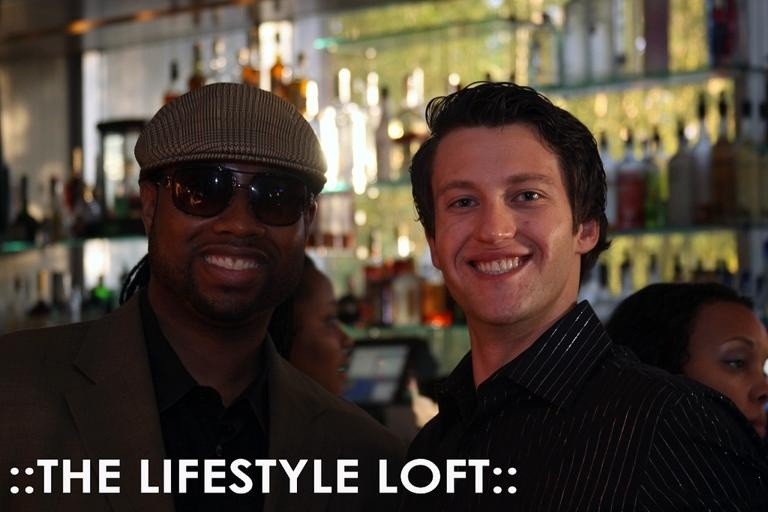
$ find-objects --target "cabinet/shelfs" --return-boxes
[0,1,767,348]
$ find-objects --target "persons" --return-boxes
[116,249,355,394]
[599,281,768,437]
[406,77,768,507]
[2,82,408,511]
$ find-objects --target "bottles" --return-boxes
[0,155,144,326]
[595,254,763,321]
[596,85,763,220]
[340,222,466,328]
[527,0,745,84]
[162,33,519,184]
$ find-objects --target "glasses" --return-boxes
[152,165,304,227]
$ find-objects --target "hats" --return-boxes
[135,81,328,188]
